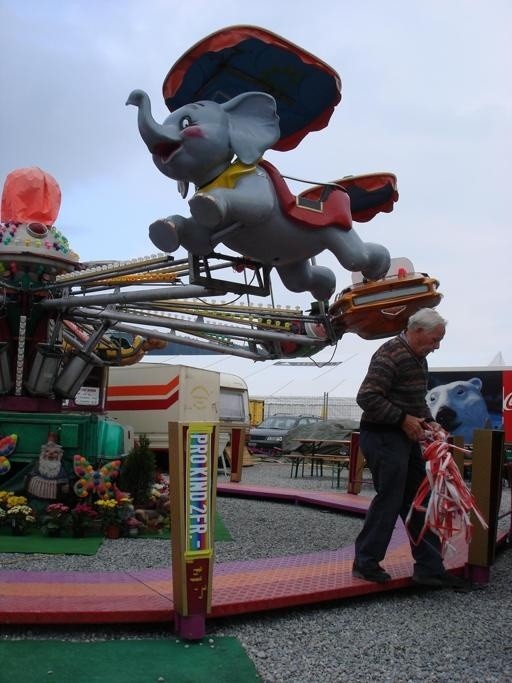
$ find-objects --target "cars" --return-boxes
[248,413,323,449]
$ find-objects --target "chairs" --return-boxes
[213,432,229,477]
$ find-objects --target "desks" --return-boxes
[300,439,352,476]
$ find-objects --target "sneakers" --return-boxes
[352,568,392,584]
[411,573,473,593]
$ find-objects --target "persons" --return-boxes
[352,304,461,589]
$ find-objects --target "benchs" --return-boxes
[281,453,368,489]
